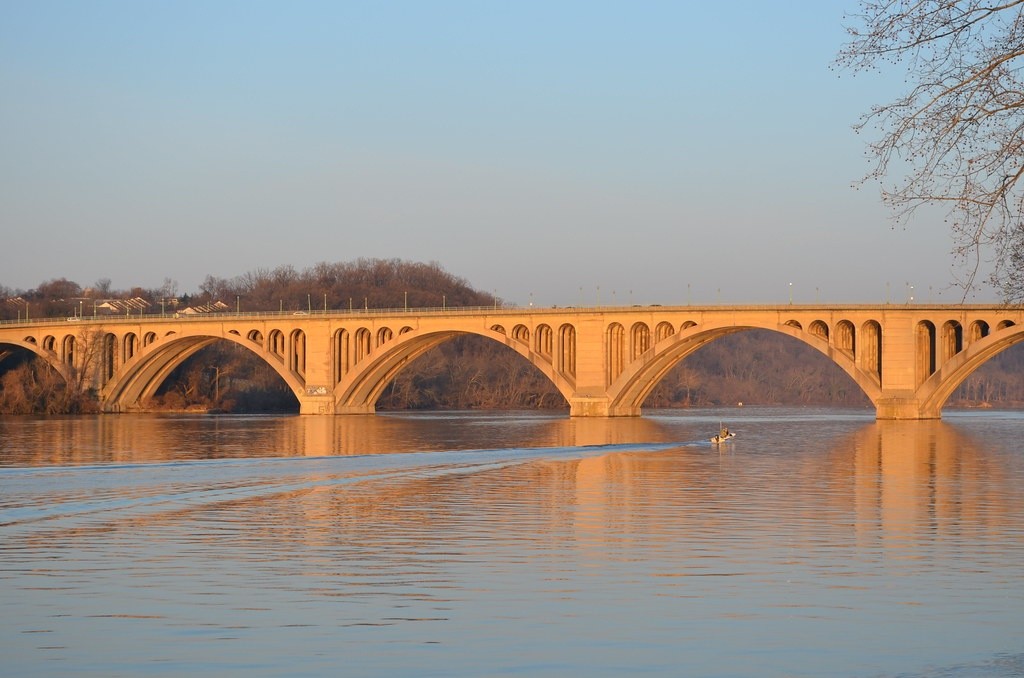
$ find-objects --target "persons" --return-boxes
[721,428,728,438]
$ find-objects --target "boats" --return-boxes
[711,432,736,444]
